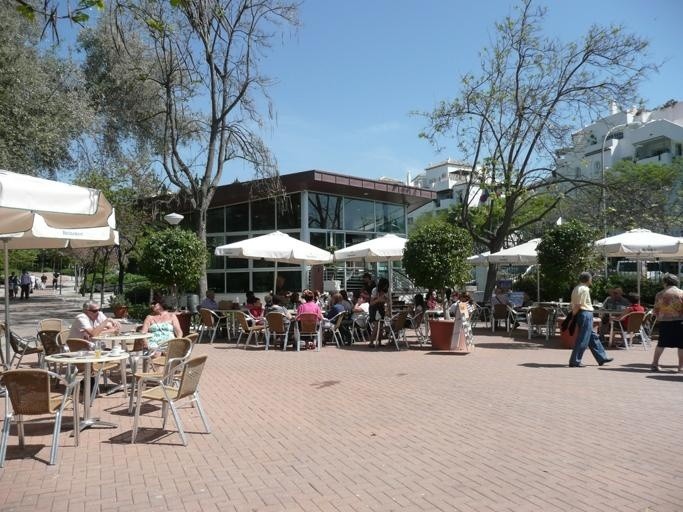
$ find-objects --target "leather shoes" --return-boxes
[598,358,612,366]
[569,363,585,367]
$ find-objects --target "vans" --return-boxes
[496,264,540,281]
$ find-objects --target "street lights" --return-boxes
[600,119,640,236]
[164,212,184,310]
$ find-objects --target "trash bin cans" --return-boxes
[187,294,199,312]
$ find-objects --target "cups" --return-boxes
[94,341,101,358]
[114,326,120,337]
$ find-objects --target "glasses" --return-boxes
[87,309,100,313]
[150,302,157,305]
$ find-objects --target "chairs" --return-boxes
[473,290,658,351]
[1,314,211,466]
[198,296,442,351]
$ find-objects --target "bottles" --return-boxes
[111,346,121,356]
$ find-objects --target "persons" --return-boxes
[491,288,520,327]
[245,278,474,350]
[599,287,629,344]
[141,296,183,359]
[8,269,60,301]
[567,271,614,367]
[201,289,228,340]
[651,273,683,372]
[613,292,644,331]
[67,300,120,392]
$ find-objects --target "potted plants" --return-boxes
[108,293,132,319]
[125,227,206,337]
[400,213,474,351]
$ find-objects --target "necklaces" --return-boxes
[152,315,170,336]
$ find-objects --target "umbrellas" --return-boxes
[625,237,683,262]
[588,228,680,305]
[214,230,334,296]
[0,207,120,369]
[0,169,113,235]
[334,233,410,318]
[487,238,544,302]
[466,248,491,266]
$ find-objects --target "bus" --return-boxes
[617,261,683,284]
